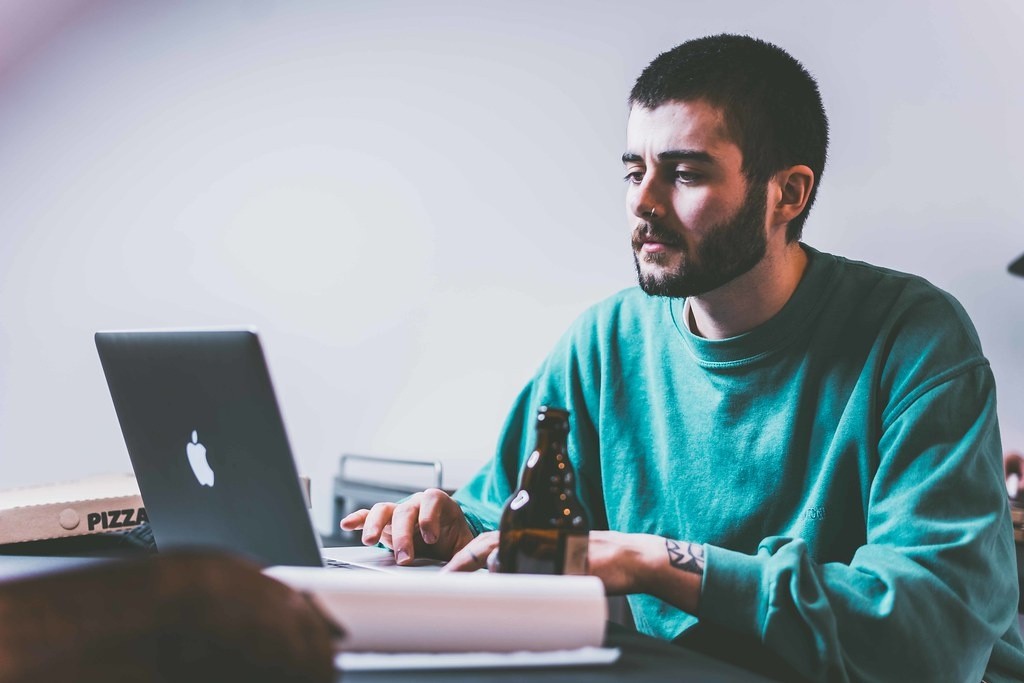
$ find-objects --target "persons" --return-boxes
[340,38,1024,683]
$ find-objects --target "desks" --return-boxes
[337,620,786,683]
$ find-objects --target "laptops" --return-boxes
[95,331,488,575]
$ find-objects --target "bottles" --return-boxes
[498,406,589,575]
